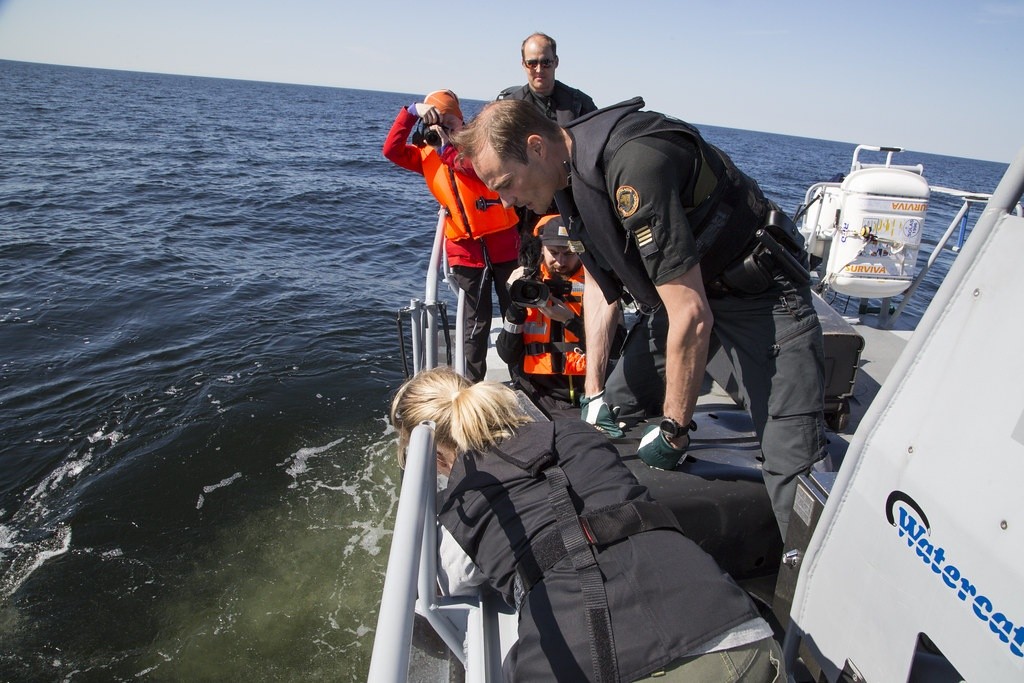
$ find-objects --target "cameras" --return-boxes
[421,123,446,147]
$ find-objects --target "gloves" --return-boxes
[638,424,691,468]
[581,390,625,437]
[505,266,536,309]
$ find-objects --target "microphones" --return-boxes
[517,235,543,270]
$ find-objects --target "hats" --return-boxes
[542,216,572,246]
[424,89,464,120]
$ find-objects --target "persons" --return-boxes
[383,32,833,544]
[390,367,789,683]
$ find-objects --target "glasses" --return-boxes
[524,58,555,68]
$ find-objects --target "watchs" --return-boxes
[564,312,574,327]
[660,416,698,438]
